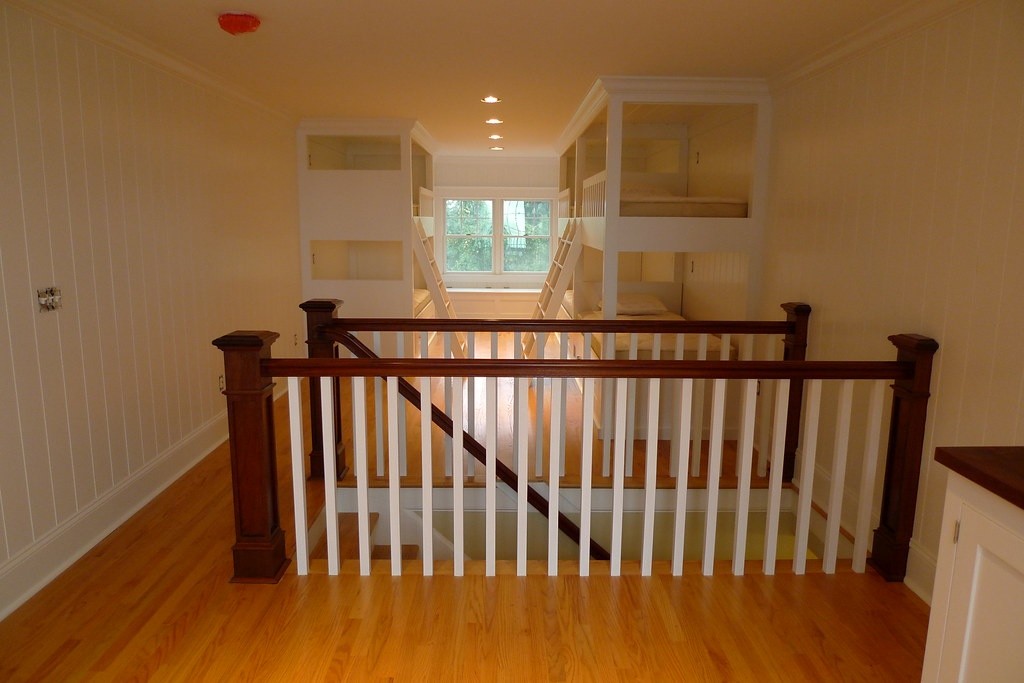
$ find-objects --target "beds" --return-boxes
[296,118,440,373]
[553,77,772,440]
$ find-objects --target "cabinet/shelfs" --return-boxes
[919,446,1024,683]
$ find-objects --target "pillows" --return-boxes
[597,292,668,315]
